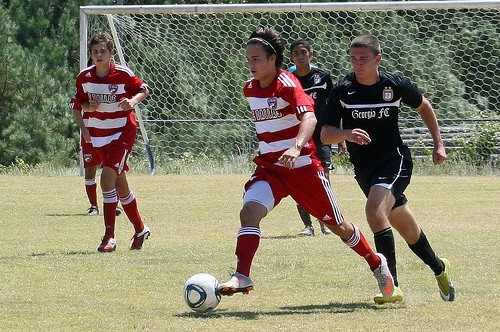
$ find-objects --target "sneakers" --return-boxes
[130,224,150,250]
[321,225,331,235]
[86,205,99,216]
[116,207,123,216]
[433,257,455,302]
[98,236,116,252]
[299,226,314,236]
[371,253,394,298]
[219,272,253,297]
[374,285,403,305]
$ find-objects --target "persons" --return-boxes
[218,26,394,297]
[289,40,333,237]
[68,36,150,252]
[320,34,455,303]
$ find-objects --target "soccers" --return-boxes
[182,273,221,313]
